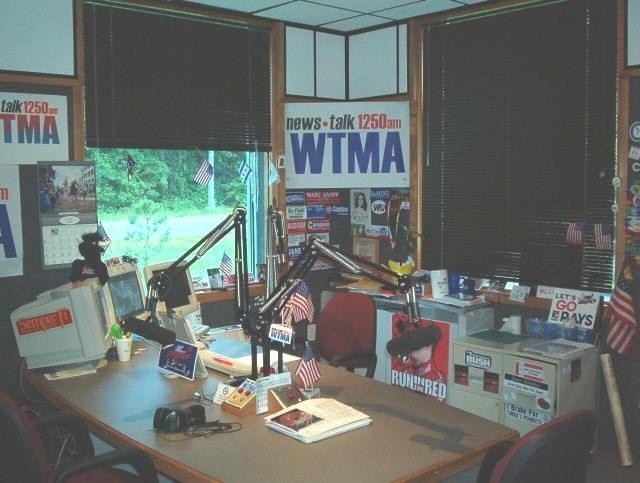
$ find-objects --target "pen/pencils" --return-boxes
[214,357,232,366]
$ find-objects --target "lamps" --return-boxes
[122,204,442,389]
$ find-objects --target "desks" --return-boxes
[479,287,552,319]
[22,332,517,481]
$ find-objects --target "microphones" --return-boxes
[412,287,420,318]
[151,291,156,316]
[145,285,152,311]
[407,292,413,325]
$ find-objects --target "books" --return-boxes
[446,292,478,302]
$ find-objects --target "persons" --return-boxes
[411,342,447,385]
[352,192,367,223]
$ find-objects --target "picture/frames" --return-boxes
[1,67,84,284]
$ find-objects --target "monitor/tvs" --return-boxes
[10,277,116,370]
[103,260,147,324]
[142,259,199,317]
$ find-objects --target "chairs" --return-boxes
[490,406,596,481]
[0,389,158,482]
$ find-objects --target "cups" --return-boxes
[115,335,132,362]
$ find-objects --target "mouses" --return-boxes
[196,341,205,349]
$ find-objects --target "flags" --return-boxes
[279,279,315,324]
[293,343,323,391]
[194,160,215,186]
[239,161,253,183]
[591,223,614,250]
[604,251,639,354]
[565,220,584,246]
[220,253,233,277]
[126,154,135,181]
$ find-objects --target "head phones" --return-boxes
[153,404,205,438]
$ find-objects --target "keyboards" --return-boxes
[200,348,258,377]
[191,324,210,335]
